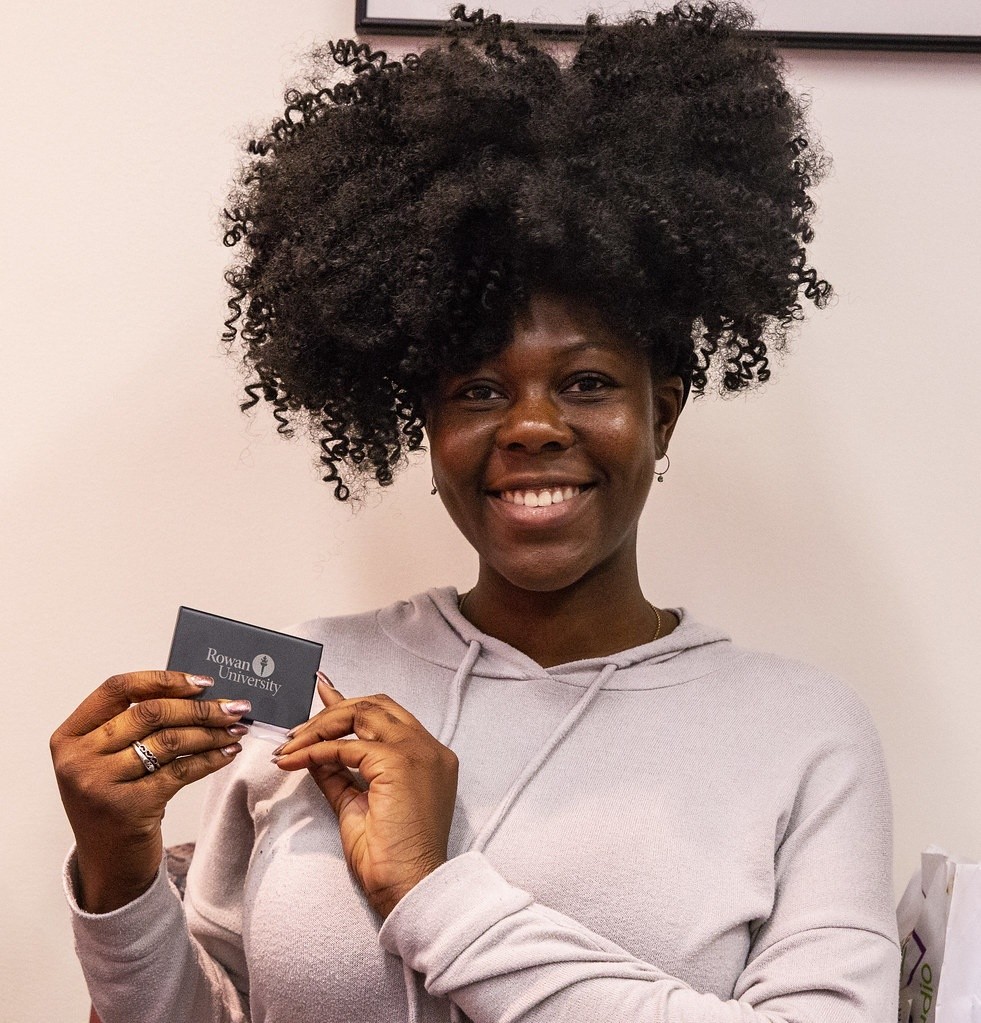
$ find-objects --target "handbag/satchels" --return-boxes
[897,843,981,1023]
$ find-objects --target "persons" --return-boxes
[50,1,902,1023]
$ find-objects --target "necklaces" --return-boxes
[458,586,662,641]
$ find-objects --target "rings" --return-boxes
[131,740,160,772]
